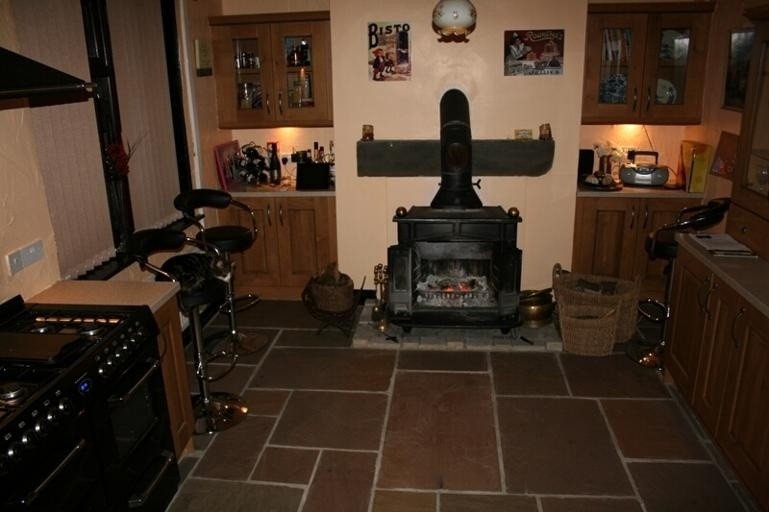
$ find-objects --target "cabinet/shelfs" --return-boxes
[212,199,336,301]
[714,290,768,470]
[660,248,739,438]
[582,5,714,124]
[209,11,332,129]
[723,32,768,262]
[154,295,197,463]
[576,196,700,304]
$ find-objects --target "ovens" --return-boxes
[3,346,182,511]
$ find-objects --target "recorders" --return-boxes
[619,151,670,187]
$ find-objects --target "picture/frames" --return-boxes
[213,139,241,193]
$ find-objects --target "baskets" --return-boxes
[555,296,623,358]
[551,261,643,346]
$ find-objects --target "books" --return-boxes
[688,229,759,261]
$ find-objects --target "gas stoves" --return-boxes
[1,300,163,437]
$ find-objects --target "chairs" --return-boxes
[127,187,267,434]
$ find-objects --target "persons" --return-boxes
[509,32,531,62]
[384,51,396,74]
[371,48,386,81]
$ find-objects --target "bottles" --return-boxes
[241,47,254,68]
[292,38,308,65]
[306,140,325,163]
[270,142,282,184]
